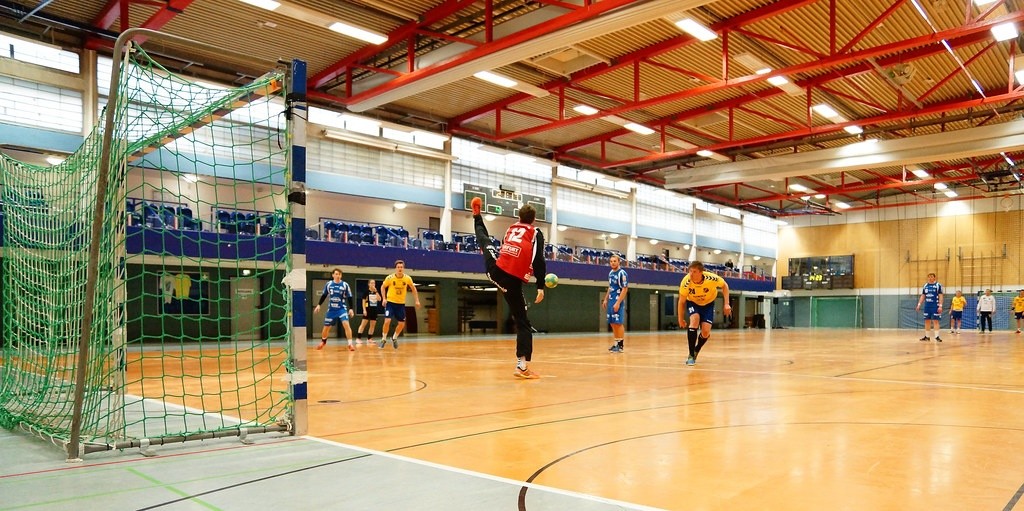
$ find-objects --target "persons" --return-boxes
[603,254,628,353]
[677,261,733,365]
[916,272,1024,342]
[312,260,421,351]
[726,259,756,274]
[471,197,546,380]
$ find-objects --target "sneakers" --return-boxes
[471,197,482,217]
[513,365,540,379]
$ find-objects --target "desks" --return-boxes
[470,320,497,333]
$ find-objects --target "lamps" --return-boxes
[239,0,1023,208]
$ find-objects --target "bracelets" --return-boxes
[918,302,921,305]
[939,305,942,308]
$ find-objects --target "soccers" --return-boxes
[545,273,559,289]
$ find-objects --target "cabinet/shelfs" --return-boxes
[428,309,438,333]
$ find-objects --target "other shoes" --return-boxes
[686,354,695,365]
[934,336,942,342]
[317,337,326,349]
[920,336,930,341]
[979,331,984,334]
[608,345,624,353]
[694,346,698,360]
[1016,330,1020,333]
[948,329,954,333]
[349,344,355,351]
[391,335,399,349]
[378,339,387,349]
[355,337,363,343]
[366,337,376,343]
[956,329,960,333]
